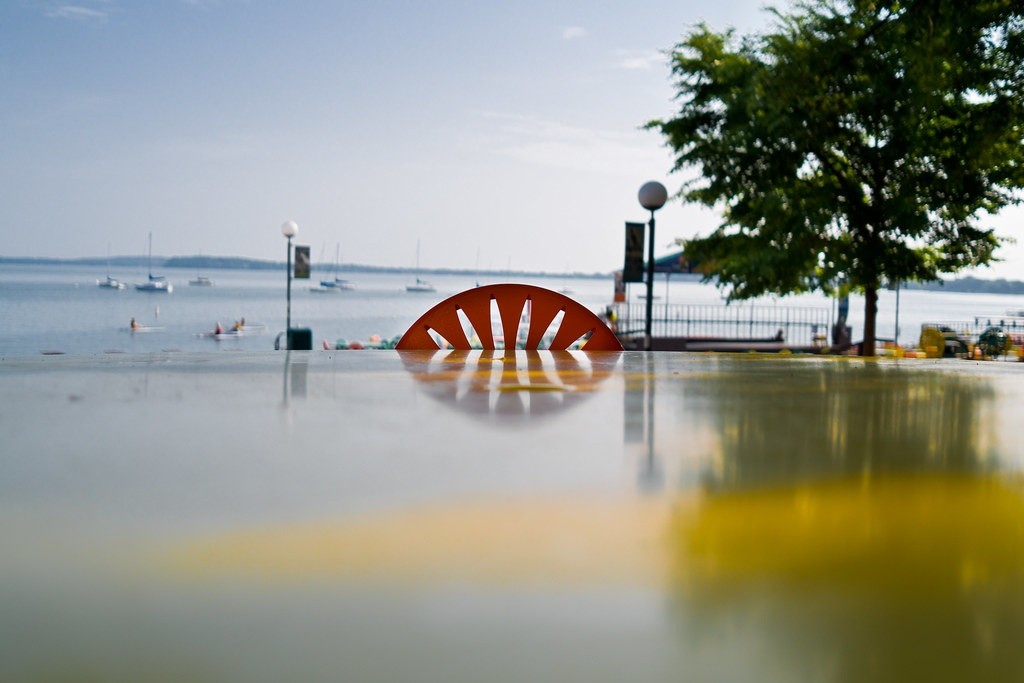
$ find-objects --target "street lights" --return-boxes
[636,180,669,351]
[279,220,299,351]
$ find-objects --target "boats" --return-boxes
[134,279,173,294]
[94,279,128,291]
[188,276,216,288]
[406,279,437,293]
[637,294,661,301]
[308,279,357,294]
